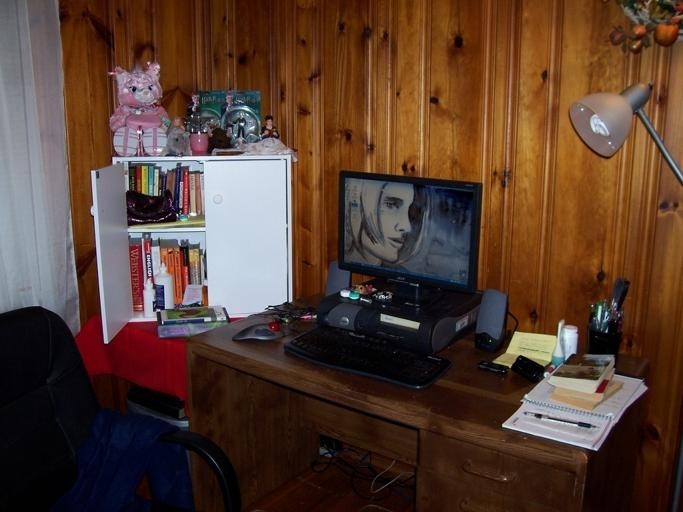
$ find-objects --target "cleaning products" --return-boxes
[142,278,157,318]
[155,262,175,312]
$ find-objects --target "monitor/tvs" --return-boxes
[338,170,484,293]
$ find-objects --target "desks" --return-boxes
[183,292,649,512]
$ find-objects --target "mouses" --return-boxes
[232,324,284,342]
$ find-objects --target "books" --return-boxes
[157,306,230,340]
[119,160,207,312]
[160,308,227,325]
[548,378,623,410]
[545,352,616,395]
[554,368,615,396]
[496,402,613,453]
[519,372,647,424]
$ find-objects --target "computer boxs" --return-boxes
[316,278,482,353]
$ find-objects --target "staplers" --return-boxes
[511,355,544,382]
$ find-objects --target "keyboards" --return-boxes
[283,324,454,390]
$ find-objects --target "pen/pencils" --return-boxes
[588,278,630,334]
[524,411,599,428]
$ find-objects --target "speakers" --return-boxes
[326,263,350,296]
[475,288,509,352]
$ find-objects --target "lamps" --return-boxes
[568,80,682,186]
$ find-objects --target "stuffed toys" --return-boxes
[106,59,172,157]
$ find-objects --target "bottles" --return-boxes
[165,116,190,155]
[143,263,175,317]
[563,324,579,362]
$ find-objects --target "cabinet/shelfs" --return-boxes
[90,155,293,344]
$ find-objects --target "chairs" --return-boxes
[0,303,242,511]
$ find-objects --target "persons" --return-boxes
[343,178,433,269]
[168,85,281,155]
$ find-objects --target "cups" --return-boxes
[588,324,622,369]
[189,133,209,155]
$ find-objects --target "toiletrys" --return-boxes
[550,318,566,366]
